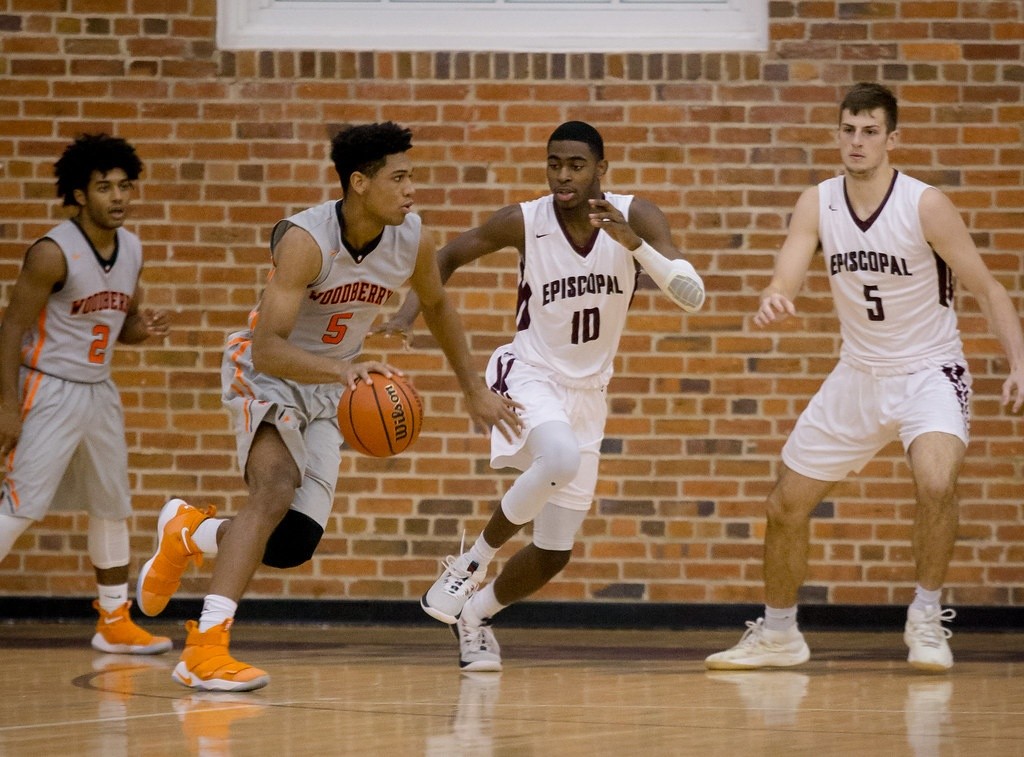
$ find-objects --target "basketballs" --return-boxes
[336,371,423,456]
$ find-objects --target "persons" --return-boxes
[705,82,1024,675]
[0,134,174,654]
[137,122,526,691]
[365,120,704,672]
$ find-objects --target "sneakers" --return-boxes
[90,598,173,655]
[172,617,269,693]
[704,616,811,669]
[901,605,957,670]
[136,498,216,616]
[448,590,502,672]
[418,551,489,625]
